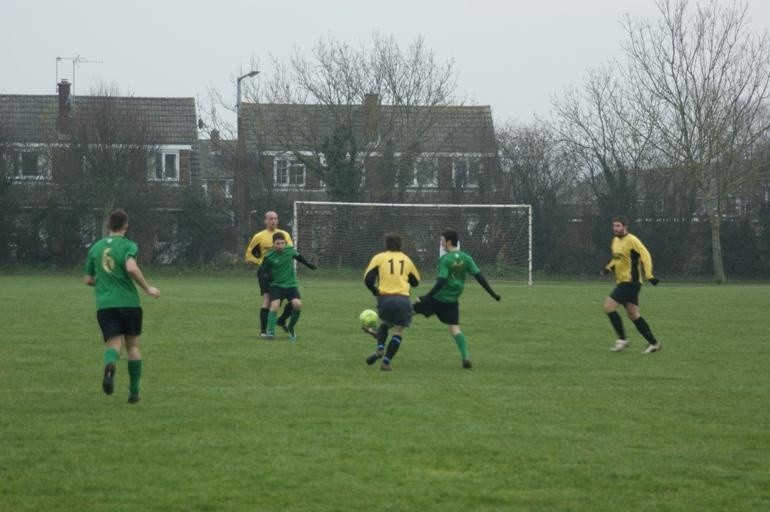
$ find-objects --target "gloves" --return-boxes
[493,294,501,302]
[309,263,316,271]
[599,267,609,277]
[649,278,658,286]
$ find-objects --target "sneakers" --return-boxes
[288,326,296,340]
[267,330,275,337]
[128,391,138,403]
[381,358,391,370]
[103,364,115,395]
[362,326,378,339]
[367,351,383,364]
[641,343,661,353]
[260,333,267,337]
[276,320,288,333]
[463,360,471,368]
[610,339,631,351]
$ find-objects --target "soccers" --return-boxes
[360,309,378,327]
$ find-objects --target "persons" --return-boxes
[440,227,460,256]
[82,208,161,403]
[363,233,421,371]
[360,230,501,368]
[245,210,293,339]
[598,216,663,354]
[258,233,317,339]
